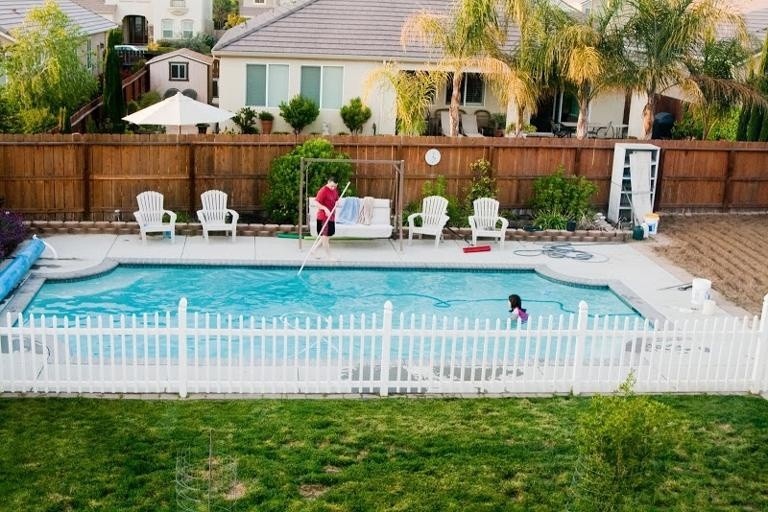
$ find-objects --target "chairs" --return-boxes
[440,111,486,137]
[132,189,240,246]
[407,195,509,248]
[550,119,571,138]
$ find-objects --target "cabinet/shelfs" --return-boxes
[606,142,661,225]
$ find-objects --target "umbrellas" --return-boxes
[121,92,239,135]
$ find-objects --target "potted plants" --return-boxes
[258,110,274,135]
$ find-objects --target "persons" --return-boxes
[311,176,344,262]
[506,293,529,320]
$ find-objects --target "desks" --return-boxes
[558,121,628,139]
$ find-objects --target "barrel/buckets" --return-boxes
[690,278,712,310]
[644,213,660,235]
